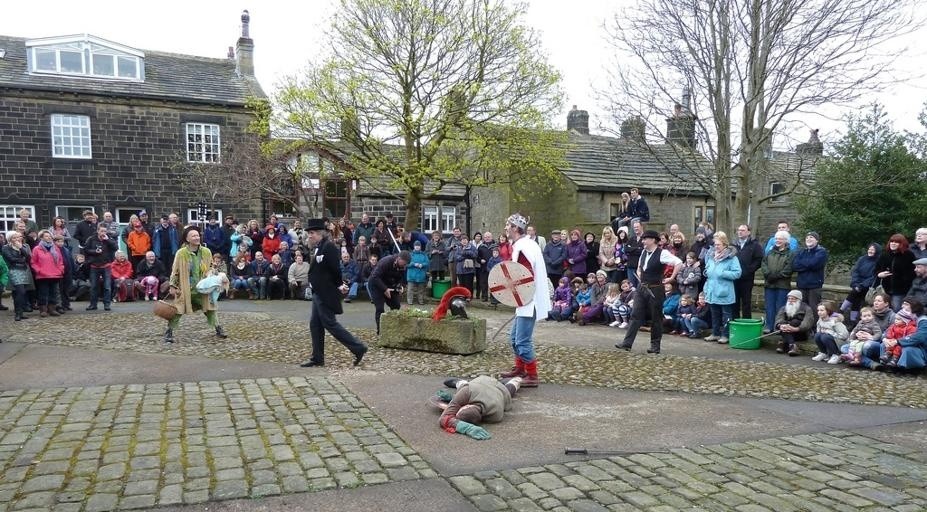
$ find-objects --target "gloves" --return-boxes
[456,421,491,440]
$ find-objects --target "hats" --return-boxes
[183,225,200,242]
[304,219,329,230]
[640,230,661,240]
[508,213,528,229]
[912,258,927,265]
[787,290,802,300]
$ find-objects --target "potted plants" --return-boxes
[377,305,486,355]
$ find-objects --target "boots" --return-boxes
[247,289,253,299]
[229,289,238,299]
[444,379,469,390]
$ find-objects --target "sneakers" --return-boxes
[600,320,628,329]
[670,331,728,343]
[502,367,538,387]
[775,344,897,367]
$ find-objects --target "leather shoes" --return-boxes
[15,305,72,320]
[353,347,367,365]
[300,361,324,366]
[647,347,660,353]
[105,303,110,310]
[86,305,96,310]
[615,343,631,351]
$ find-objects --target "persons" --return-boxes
[164,225,228,341]
[700,220,926,375]
[617,188,652,230]
[500,212,553,389]
[202,208,712,338]
[437,373,524,441]
[303,219,369,367]
[3,207,188,322]
[615,231,682,353]
[611,191,630,231]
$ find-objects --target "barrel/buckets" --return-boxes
[727,315,765,350]
[431,278,450,299]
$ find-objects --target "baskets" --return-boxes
[154,292,177,320]
